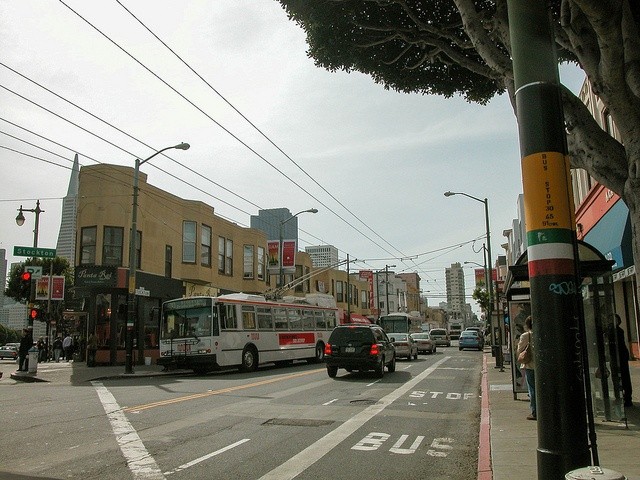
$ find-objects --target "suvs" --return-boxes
[324,324,396,377]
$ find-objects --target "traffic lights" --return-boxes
[31,308,41,319]
[21,272,31,298]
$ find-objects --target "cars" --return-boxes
[410,333,436,354]
[459,331,483,350]
[429,329,450,347]
[386,333,418,360]
[0,346,18,360]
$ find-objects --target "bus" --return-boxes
[380,313,423,333]
[159,292,340,372]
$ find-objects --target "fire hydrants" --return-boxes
[26,347,39,374]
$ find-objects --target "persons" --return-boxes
[604,312,635,408]
[36,329,81,364]
[16,328,29,371]
[88,332,98,367]
[517,315,536,420]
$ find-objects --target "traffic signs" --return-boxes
[25,266,42,279]
[14,246,56,258]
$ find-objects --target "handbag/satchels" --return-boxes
[517,331,531,364]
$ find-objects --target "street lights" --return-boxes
[16,200,45,304]
[444,191,494,313]
[126,142,190,373]
[464,261,488,291]
[280,208,318,288]
